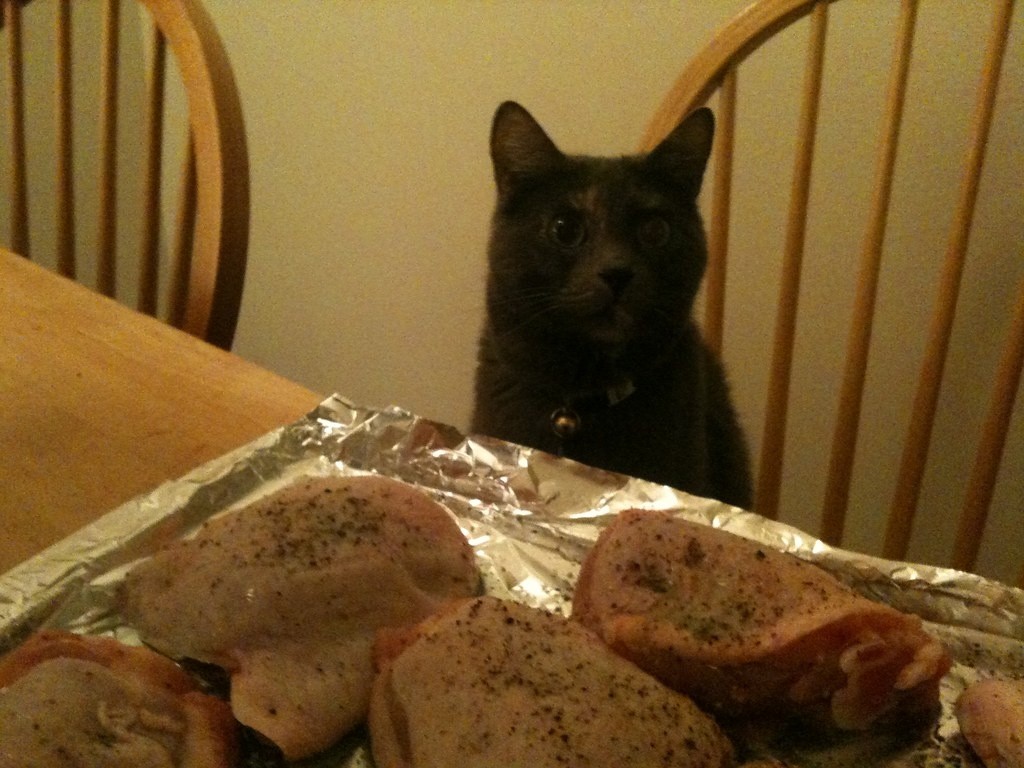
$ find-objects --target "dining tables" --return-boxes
[0,245,321,577]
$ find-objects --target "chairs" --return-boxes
[0,2,252,349]
[642,0,1023,587]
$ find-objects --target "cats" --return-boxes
[470,96,754,508]
